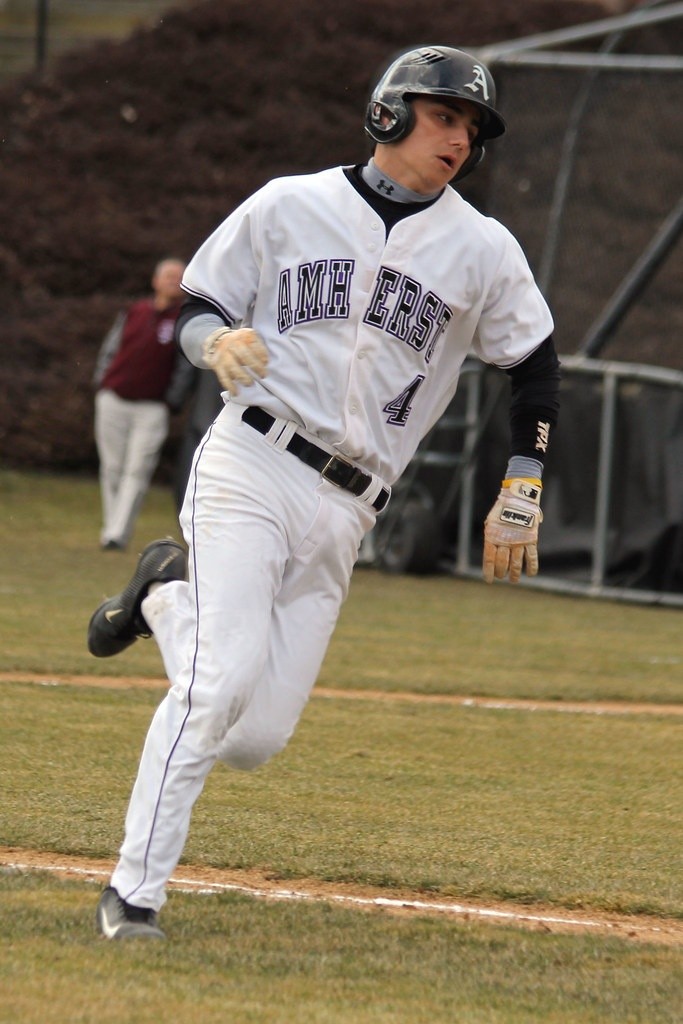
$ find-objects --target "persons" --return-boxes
[92,254,203,549]
[88,45,562,938]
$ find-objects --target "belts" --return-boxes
[242,405,390,511]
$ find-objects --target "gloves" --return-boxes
[481,479,544,584]
[201,326,269,396]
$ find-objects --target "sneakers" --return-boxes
[87,538,189,658]
[94,886,168,941]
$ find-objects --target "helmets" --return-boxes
[363,46,508,182]
